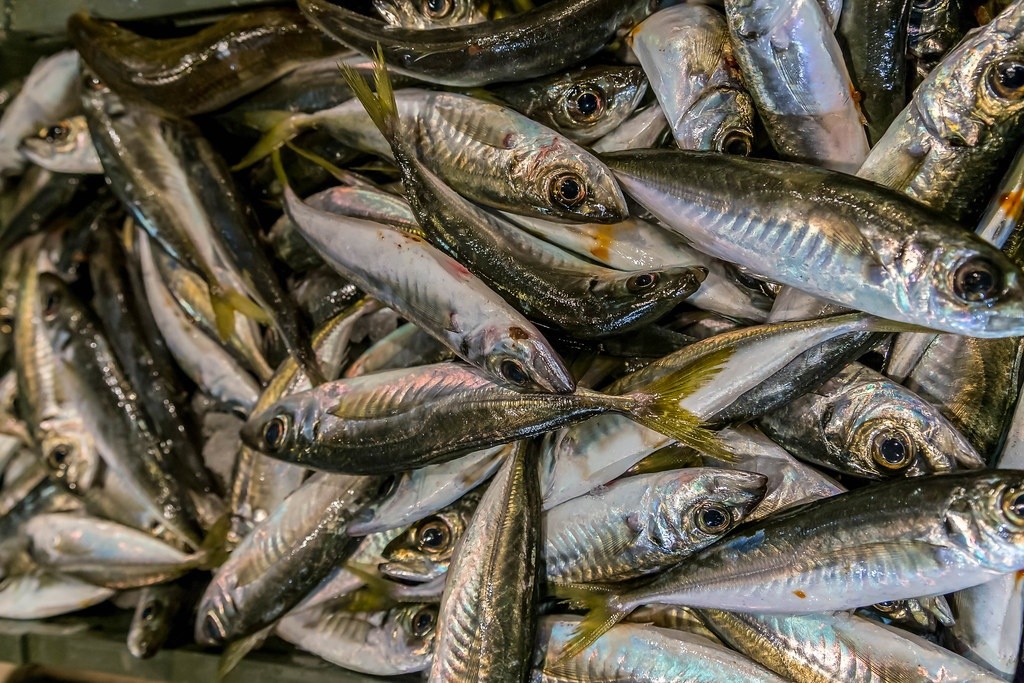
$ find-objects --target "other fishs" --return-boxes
[2,0,1024,683]
